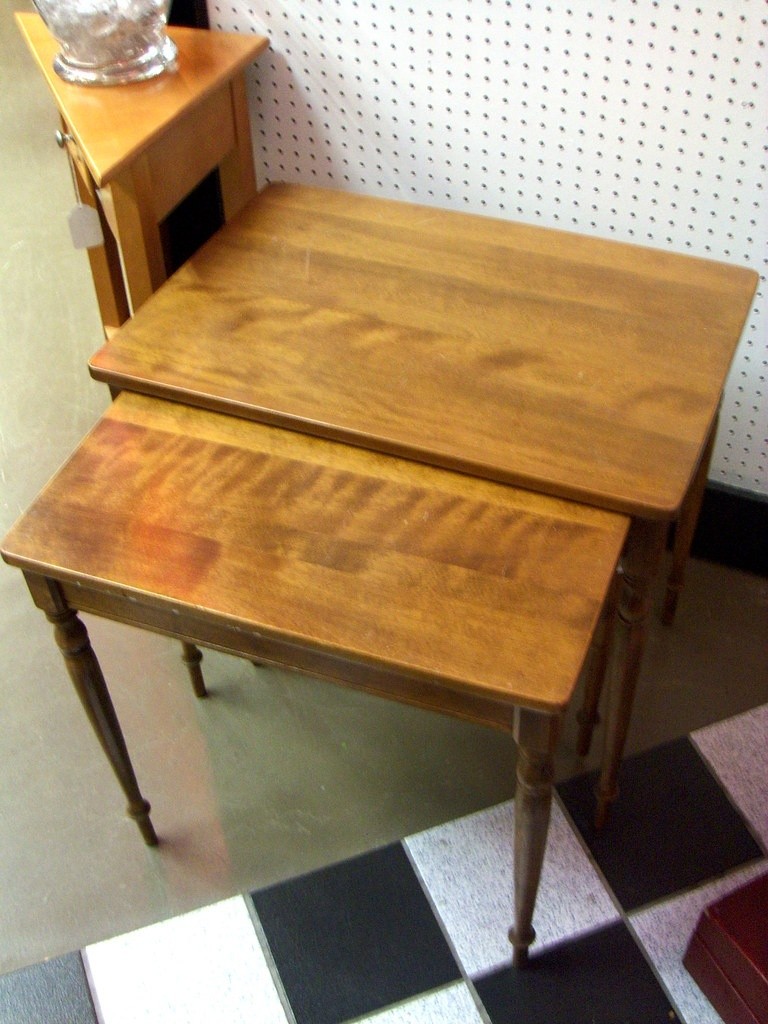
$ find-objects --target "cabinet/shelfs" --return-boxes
[14,10,270,401]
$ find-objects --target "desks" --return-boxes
[88,182,759,831]
[0,389,631,965]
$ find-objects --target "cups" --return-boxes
[34,0,181,88]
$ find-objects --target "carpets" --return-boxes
[0,702,767,1024]
